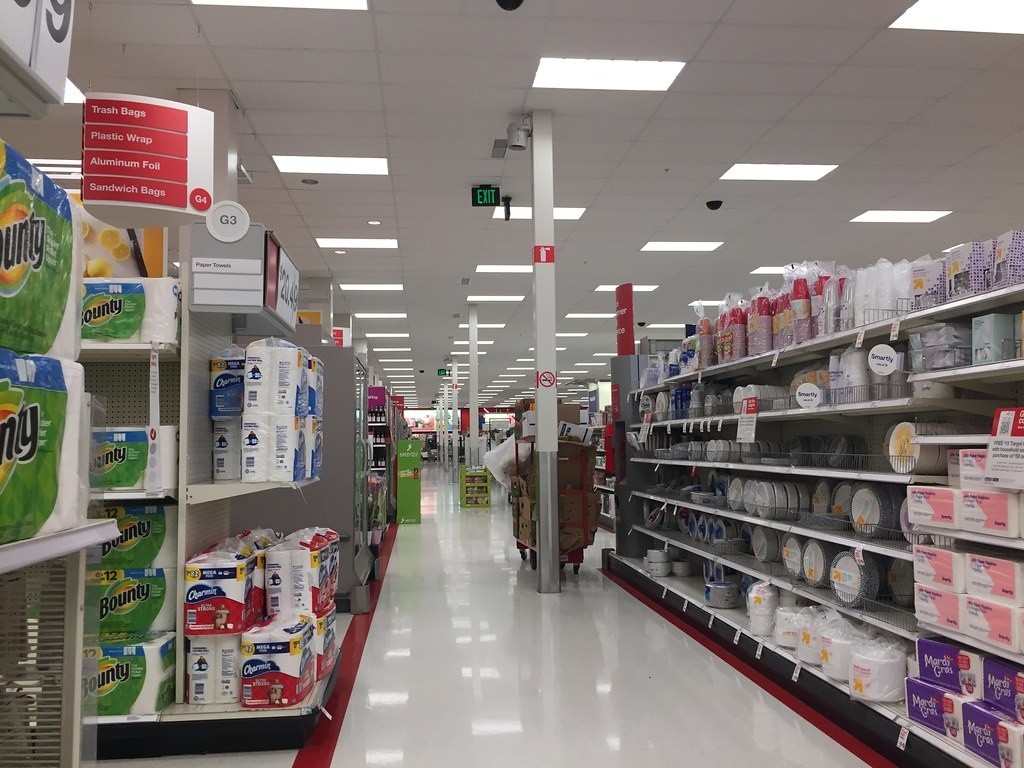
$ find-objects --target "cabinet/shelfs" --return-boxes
[601,285,1024,768]
[587,425,617,533]
[0,142,396,768]
[460,466,491,508]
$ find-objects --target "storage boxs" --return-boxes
[515,401,602,554]
[909,230,1024,365]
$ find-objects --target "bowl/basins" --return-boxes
[654,448,669,459]
[643,546,690,578]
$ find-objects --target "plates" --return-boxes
[704,385,791,416]
[639,392,670,423]
[688,435,866,469]
[796,383,821,408]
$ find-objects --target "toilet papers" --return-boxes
[0,141,340,716]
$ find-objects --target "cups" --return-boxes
[828,350,903,403]
[853,261,910,328]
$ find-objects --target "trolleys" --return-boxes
[513,441,590,575]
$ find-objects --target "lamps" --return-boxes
[505,122,532,151]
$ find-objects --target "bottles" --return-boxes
[368,405,385,423]
[372,450,386,467]
[372,430,384,444]
